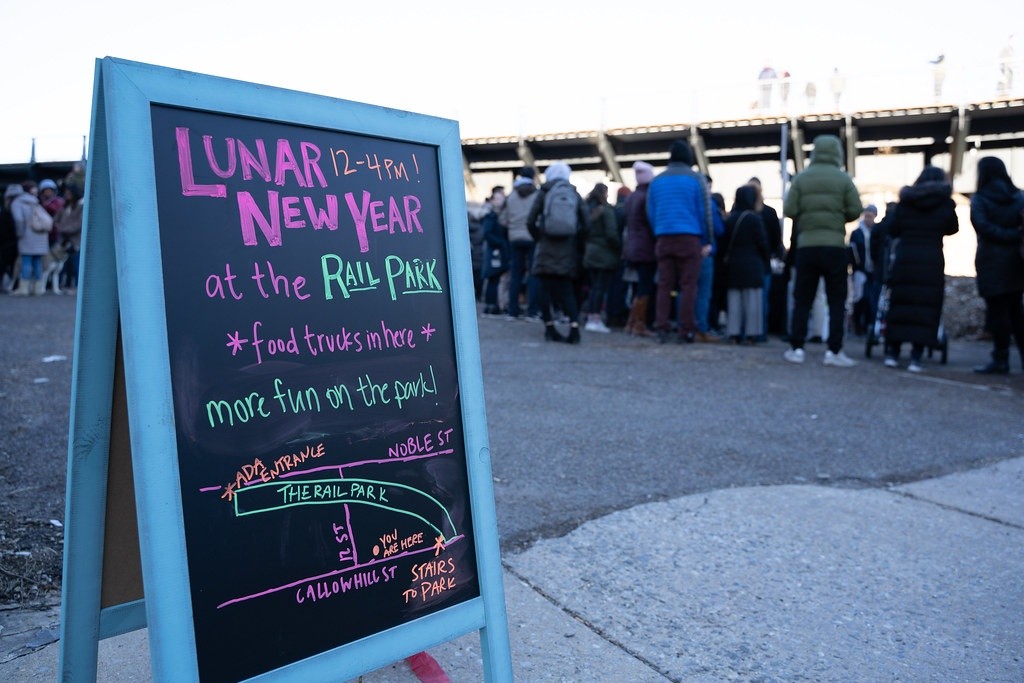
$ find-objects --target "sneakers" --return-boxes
[823,350,858,367]
[782,347,805,364]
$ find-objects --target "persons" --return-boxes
[701,174,790,346]
[879,167,959,373]
[466,133,723,345]
[0,164,84,297]
[969,157,1024,376]
[847,202,898,338]
[784,130,861,367]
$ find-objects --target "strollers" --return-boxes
[864,234,948,364]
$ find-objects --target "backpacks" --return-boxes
[542,181,577,235]
[21,200,53,233]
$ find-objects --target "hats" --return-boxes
[670,140,696,166]
[4,184,23,195]
[633,161,654,184]
[863,205,879,217]
[519,168,534,181]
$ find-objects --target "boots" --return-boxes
[625,299,656,336]
[10,279,76,298]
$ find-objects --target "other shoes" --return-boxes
[975,362,1009,375]
[884,357,898,367]
[694,331,719,344]
[730,334,744,344]
[585,320,610,333]
[909,360,927,372]
[543,325,566,341]
[746,334,767,346]
[480,306,569,325]
[658,330,672,344]
[678,333,694,343]
[566,326,581,344]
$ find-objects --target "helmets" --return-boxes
[38,180,57,193]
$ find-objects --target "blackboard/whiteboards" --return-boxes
[60,56,505,683]
